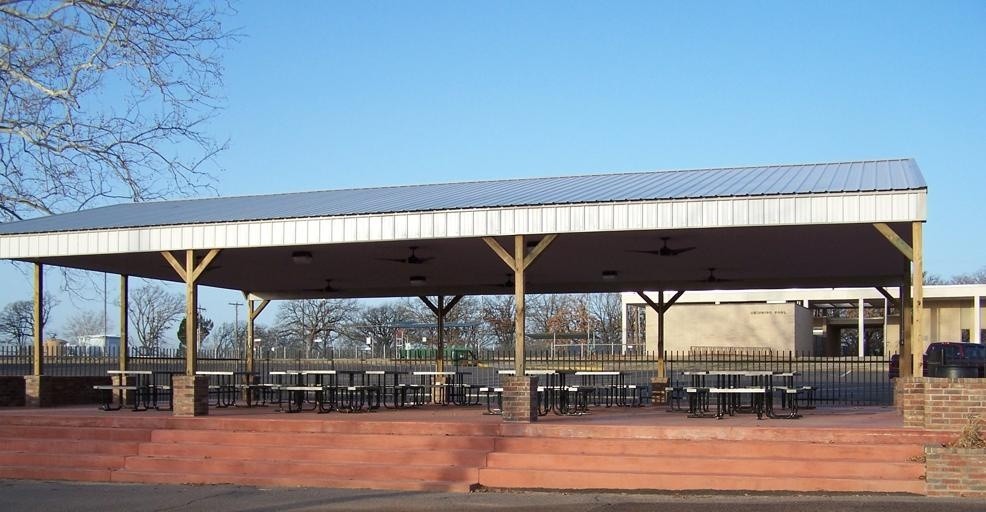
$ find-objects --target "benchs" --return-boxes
[89,384,172,417]
[530,383,824,418]
[209,383,502,413]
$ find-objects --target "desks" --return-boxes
[195,369,473,418]
[496,369,801,416]
[108,370,173,412]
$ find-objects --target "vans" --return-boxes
[928,342,985,377]
[889,354,928,378]
